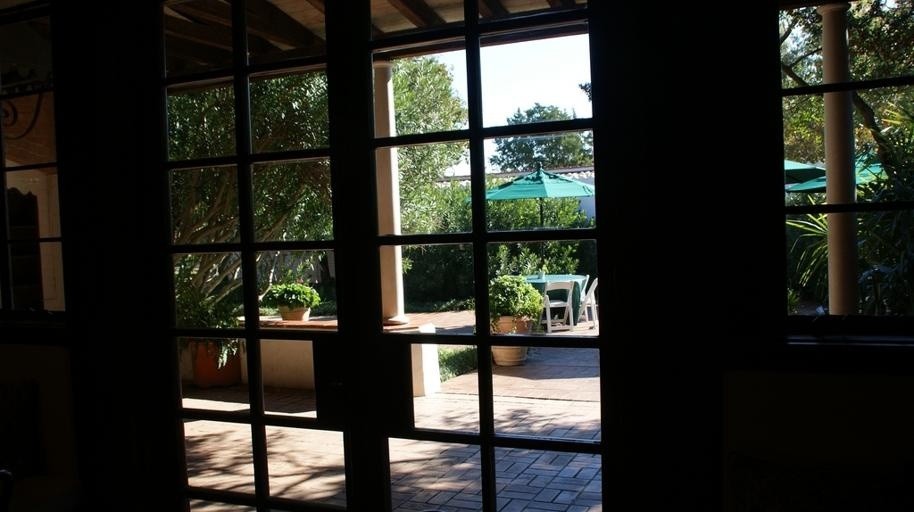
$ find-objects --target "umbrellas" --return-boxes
[786,147,888,193]
[464,161,594,270]
[785,157,827,185]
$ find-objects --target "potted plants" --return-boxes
[268,283,321,321]
[488,275,546,367]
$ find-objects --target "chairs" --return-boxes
[535,278,598,331]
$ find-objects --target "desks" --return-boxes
[524,274,589,324]
